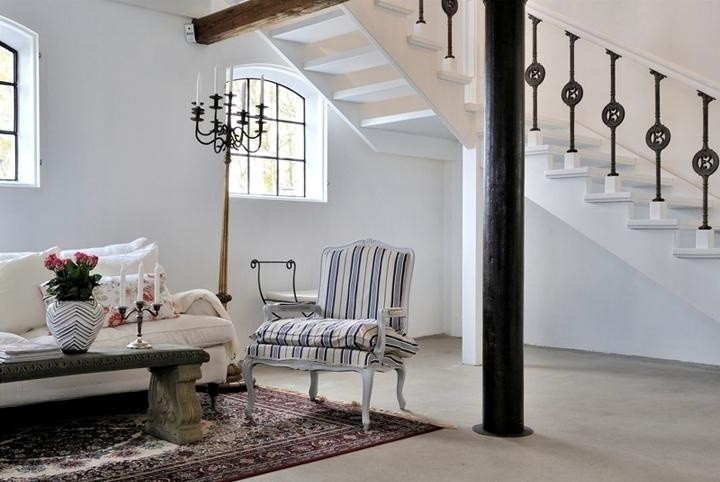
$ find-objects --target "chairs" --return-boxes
[243,238,421,433]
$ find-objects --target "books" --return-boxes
[0,340,65,365]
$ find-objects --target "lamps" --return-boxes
[190,62,267,395]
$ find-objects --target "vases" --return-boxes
[46,296,105,355]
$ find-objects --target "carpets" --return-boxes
[1,383,445,482]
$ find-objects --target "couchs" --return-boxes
[0,237,236,409]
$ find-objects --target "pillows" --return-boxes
[0,241,181,339]
